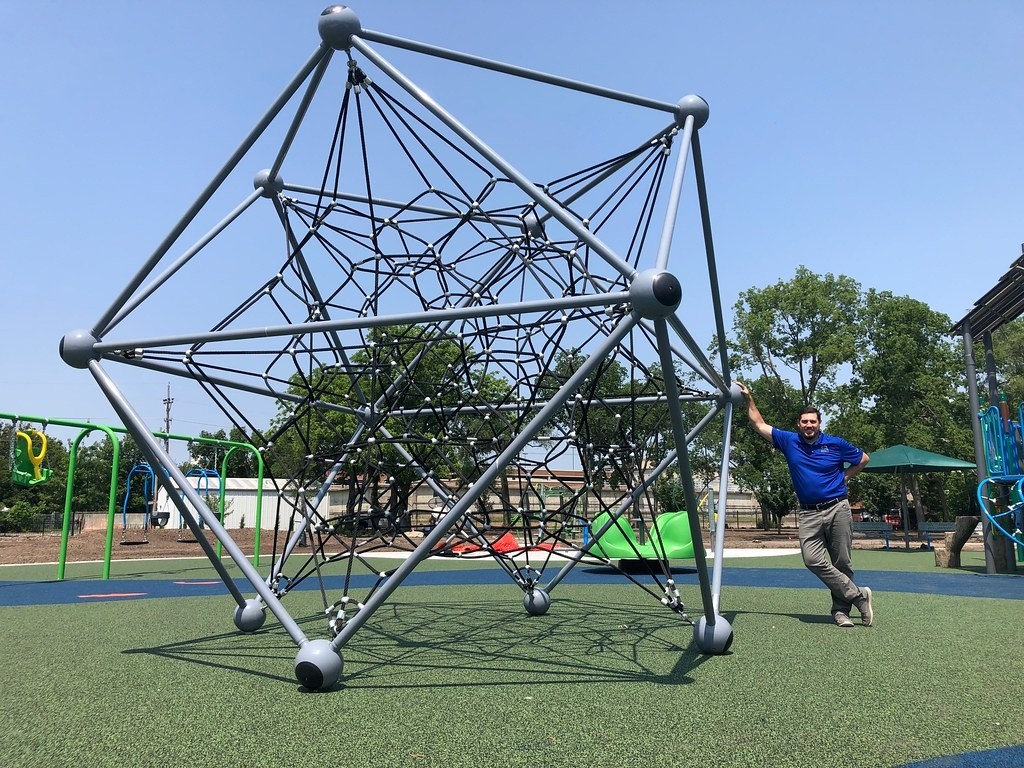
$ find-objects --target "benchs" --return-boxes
[918,522,955,551]
[852,522,900,550]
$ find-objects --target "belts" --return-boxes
[800,495,848,512]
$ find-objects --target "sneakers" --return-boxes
[832,611,854,627]
[858,587,874,627]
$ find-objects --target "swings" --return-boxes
[177,435,222,543]
[7,414,54,486]
[119,432,172,546]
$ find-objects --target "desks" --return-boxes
[630,518,653,546]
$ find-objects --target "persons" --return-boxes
[735,381,873,627]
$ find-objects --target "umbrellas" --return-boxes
[844,444,978,549]
